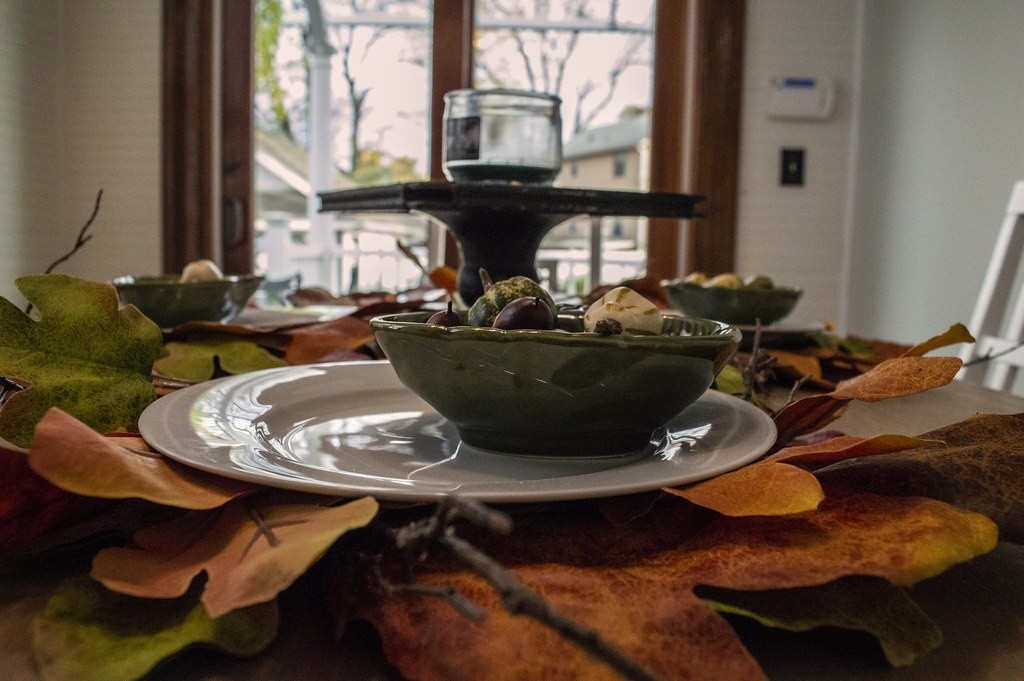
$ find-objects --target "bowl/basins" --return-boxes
[442,88,562,188]
[371,312,740,456]
[659,278,802,326]
[116,273,265,332]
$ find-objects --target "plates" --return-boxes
[137,359,777,499]
[741,322,827,335]
